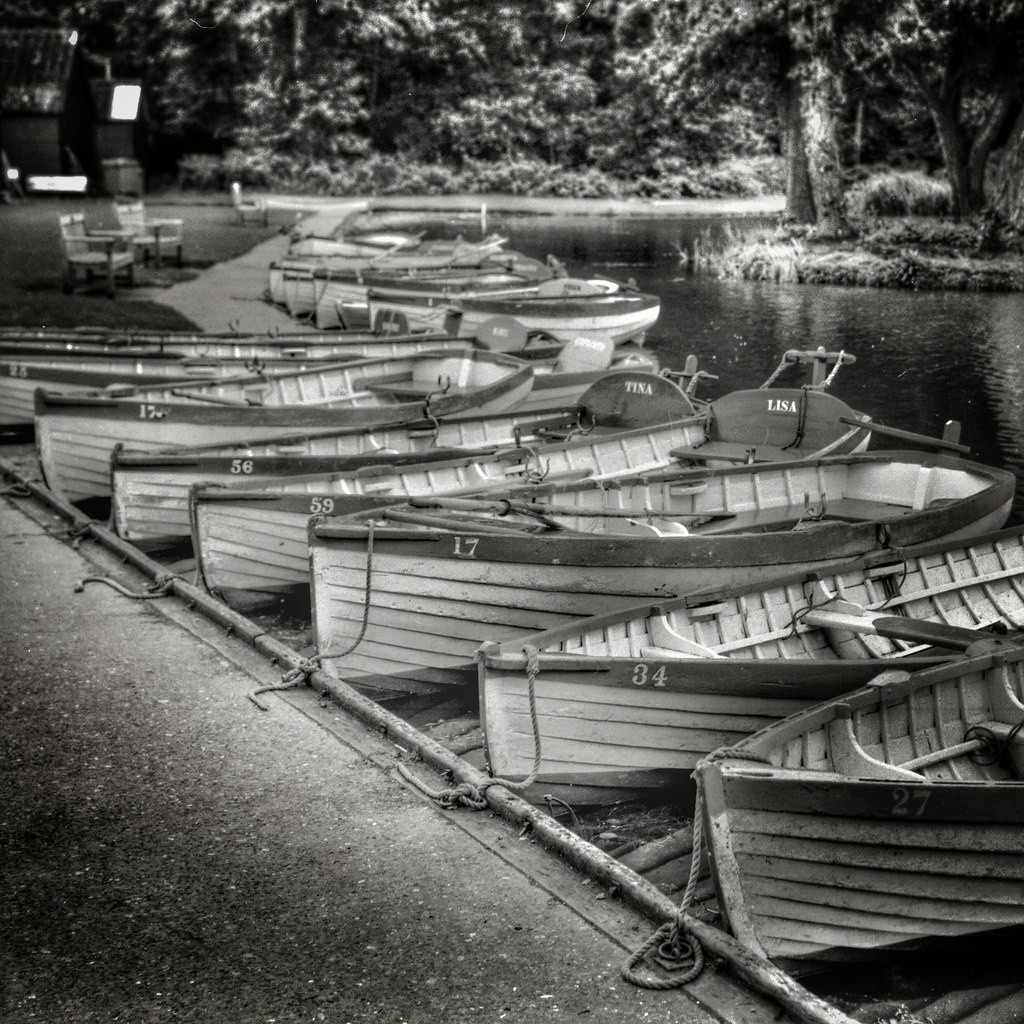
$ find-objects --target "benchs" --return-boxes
[798,600,1007,650]
[367,379,483,398]
[682,497,911,536]
[672,439,817,463]
[532,424,632,439]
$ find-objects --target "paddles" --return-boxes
[407,488,734,520]
[370,229,427,267]
[185,389,265,413]
[448,235,510,272]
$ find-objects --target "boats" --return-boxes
[474,525,1023,828]
[297,412,1019,685]
[691,639,1023,980]
[178,346,868,618]
[0,229,680,559]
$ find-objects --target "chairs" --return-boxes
[55,206,138,299]
[111,198,185,271]
[230,182,270,228]
